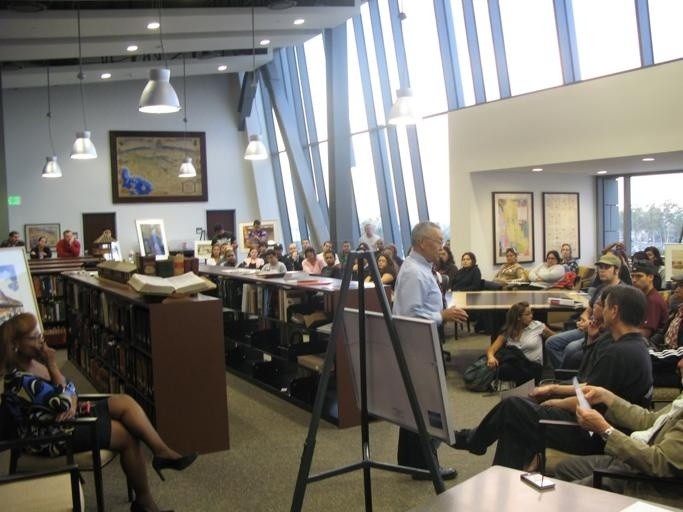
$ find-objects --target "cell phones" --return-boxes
[520,470,555,491]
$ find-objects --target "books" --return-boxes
[217,276,334,334]
[128,271,217,297]
[32,275,154,428]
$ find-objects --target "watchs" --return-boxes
[598,427,613,442]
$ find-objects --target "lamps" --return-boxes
[387,0,422,125]
[178,55,197,177]
[42,66,62,178]
[138,9,182,115]
[70,8,97,158]
[244,8,268,162]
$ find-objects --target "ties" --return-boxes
[432,266,447,308]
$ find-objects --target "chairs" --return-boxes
[648,360,683,407]
[7,393,133,512]
[537,378,654,474]
[594,469,681,499]
[1,430,86,512]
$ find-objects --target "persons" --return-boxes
[0,312,200,512]
[449,286,652,472]
[2,229,81,261]
[554,357,683,505]
[431,246,683,389]
[391,221,468,479]
[207,219,403,291]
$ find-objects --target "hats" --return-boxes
[595,255,621,268]
[632,262,656,274]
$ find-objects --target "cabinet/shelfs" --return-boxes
[29,262,84,346]
[199,267,392,429]
[60,272,231,456]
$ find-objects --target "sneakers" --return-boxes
[490,379,516,391]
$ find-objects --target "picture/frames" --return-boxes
[136,218,170,261]
[239,221,277,252]
[195,240,213,259]
[543,192,580,262]
[24,224,61,253]
[492,191,535,265]
[109,130,208,202]
[664,242,683,282]
[0,247,44,334]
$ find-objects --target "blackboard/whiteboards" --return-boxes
[340,306,456,446]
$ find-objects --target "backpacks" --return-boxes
[464,355,499,391]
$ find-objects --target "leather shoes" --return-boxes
[450,428,486,455]
[414,466,456,480]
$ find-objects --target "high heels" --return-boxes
[152,452,198,481]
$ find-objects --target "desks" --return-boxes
[449,288,594,361]
[403,465,683,512]
